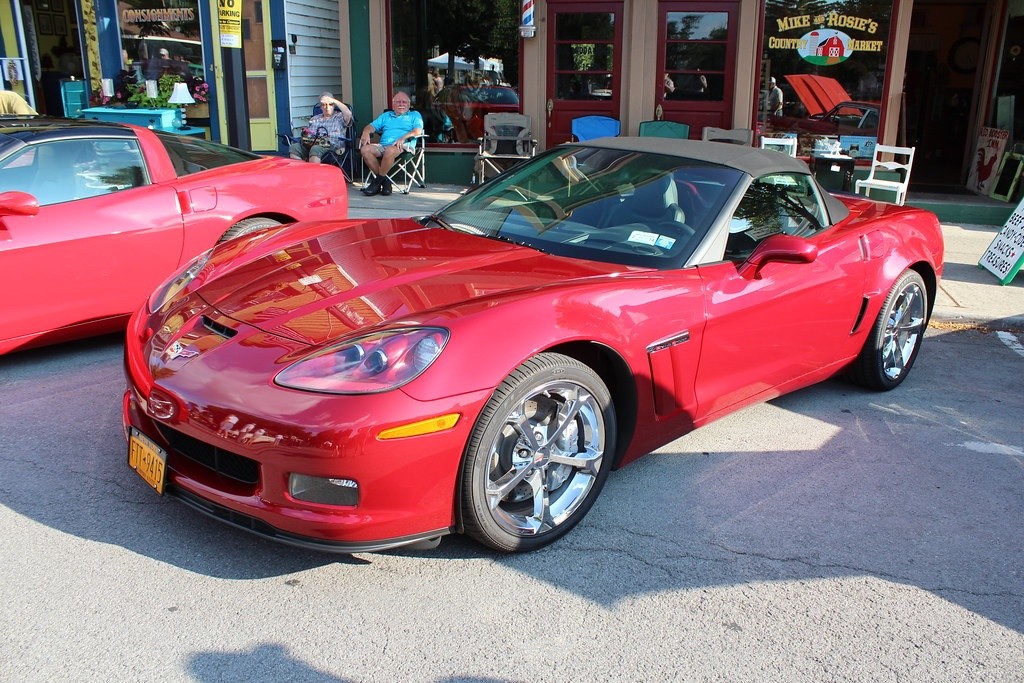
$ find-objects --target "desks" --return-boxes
[809,153,855,195]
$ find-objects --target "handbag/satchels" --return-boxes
[301,128,330,147]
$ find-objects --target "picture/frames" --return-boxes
[989,152,1024,203]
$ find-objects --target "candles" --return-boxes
[102,78,114,96]
[146,80,158,99]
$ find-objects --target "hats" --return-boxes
[769,77,776,84]
[159,48,169,55]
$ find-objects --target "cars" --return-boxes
[0,118,349,357]
[429,83,519,137]
[772,74,884,169]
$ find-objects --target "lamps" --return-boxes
[168,82,195,131]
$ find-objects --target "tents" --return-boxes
[428,49,506,80]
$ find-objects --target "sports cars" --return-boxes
[121,137,950,550]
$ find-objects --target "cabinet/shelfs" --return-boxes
[81,106,206,140]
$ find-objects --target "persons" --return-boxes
[427,65,506,101]
[0,90,39,119]
[360,91,424,196]
[662,68,707,98]
[121,48,198,86]
[769,77,784,117]
[288,92,352,163]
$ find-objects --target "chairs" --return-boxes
[636,120,689,185]
[568,115,621,197]
[702,127,753,148]
[358,108,429,194]
[277,103,356,183]
[854,143,916,206]
[474,113,538,205]
[760,135,798,187]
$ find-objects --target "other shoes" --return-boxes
[381,181,392,195]
[363,179,381,196]
